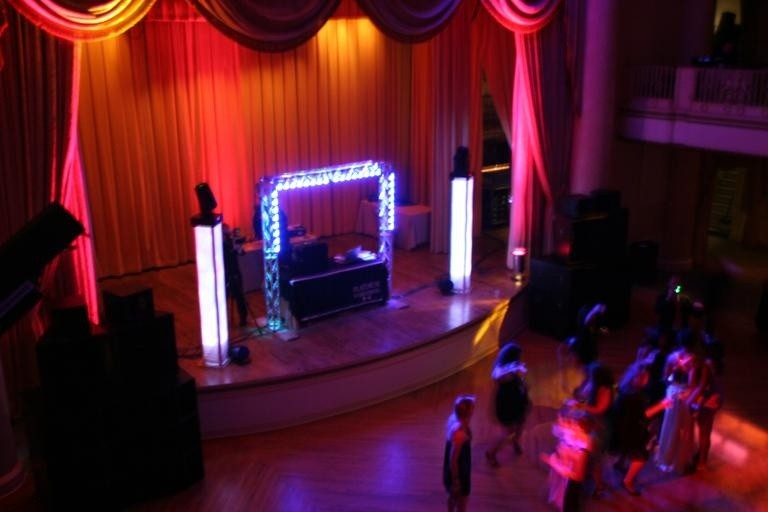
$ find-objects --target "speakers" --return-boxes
[196,183,216,211]
[453,146,469,176]
[0,200,85,336]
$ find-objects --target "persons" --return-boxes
[444,397,476,512]
[565,273,725,500]
[485,343,532,464]
[222,224,247,327]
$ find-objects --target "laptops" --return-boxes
[334,245,362,265]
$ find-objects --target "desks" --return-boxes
[234,233,321,295]
[359,197,432,252]
[282,255,390,329]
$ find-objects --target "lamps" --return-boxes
[192,218,233,369]
[447,176,475,295]
